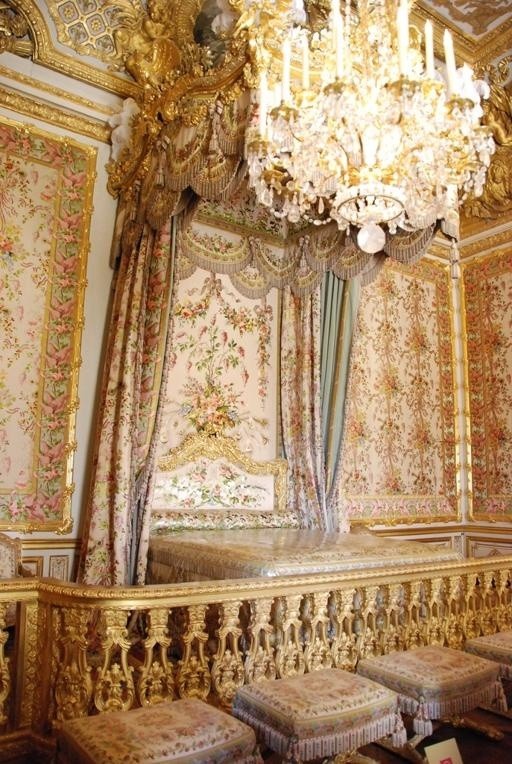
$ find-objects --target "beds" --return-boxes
[143,423,454,587]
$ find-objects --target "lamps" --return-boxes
[243,0,498,257]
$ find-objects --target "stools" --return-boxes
[355,644,502,764]
[463,625,512,681]
[236,666,400,764]
[55,698,258,762]
